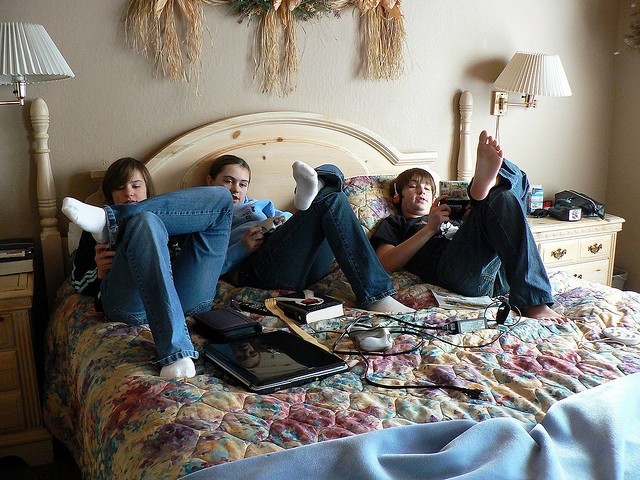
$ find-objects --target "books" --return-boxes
[275,288,345,325]
[0,238,34,276]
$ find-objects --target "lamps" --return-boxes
[491,50,573,117]
[0,20,74,107]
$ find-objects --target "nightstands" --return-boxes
[526,212,626,287]
[0,269,57,467]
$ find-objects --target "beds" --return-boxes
[30,90,640,480]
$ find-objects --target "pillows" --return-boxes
[341,173,400,239]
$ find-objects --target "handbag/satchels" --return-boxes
[555,190,605,220]
[192,307,262,344]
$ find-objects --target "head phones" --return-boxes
[392,168,436,205]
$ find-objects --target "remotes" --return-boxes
[231,298,273,316]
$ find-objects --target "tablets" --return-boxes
[438,199,471,223]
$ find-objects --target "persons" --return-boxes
[206,155,417,315]
[231,341,261,369]
[61,157,234,381]
[369,130,570,324]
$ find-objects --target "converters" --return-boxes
[451,317,488,334]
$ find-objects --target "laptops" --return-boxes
[203,330,351,396]
